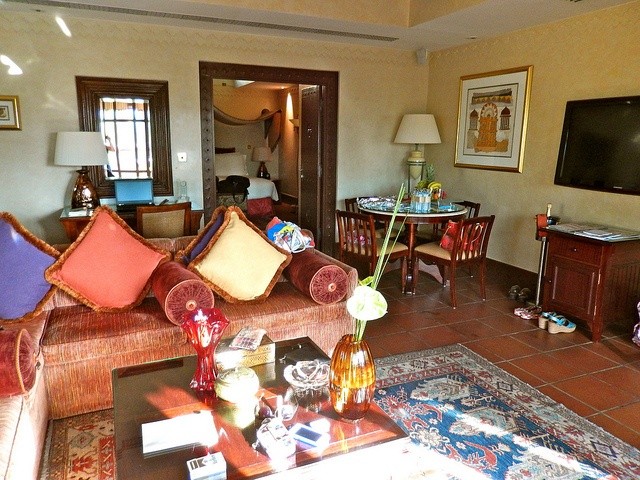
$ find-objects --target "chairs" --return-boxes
[335,208,409,294]
[414,199,481,243]
[345,197,406,241]
[409,215,495,310]
[135,202,192,238]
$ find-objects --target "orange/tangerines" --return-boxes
[433,193,441,200]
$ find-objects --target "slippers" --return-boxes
[515,305,543,317]
[521,312,542,319]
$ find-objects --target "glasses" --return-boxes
[252,393,299,421]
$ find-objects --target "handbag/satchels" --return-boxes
[217,174,250,203]
[440,219,486,252]
[266,216,316,254]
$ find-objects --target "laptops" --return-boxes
[115,179,156,214]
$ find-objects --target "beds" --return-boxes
[215,147,280,222]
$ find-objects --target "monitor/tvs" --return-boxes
[555,96,636,196]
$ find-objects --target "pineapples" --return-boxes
[427,164,437,187]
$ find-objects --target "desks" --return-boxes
[60,208,209,243]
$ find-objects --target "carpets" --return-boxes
[40,342,639,478]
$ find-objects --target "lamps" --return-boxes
[392,114,442,195]
[252,146,273,179]
[53,131,109,211]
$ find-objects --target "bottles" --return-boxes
[410,188,433,211]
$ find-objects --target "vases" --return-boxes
[329,335,375,422]
[181,306,230,392]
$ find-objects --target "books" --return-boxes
[547,222,640,240]
[141,410,219,460]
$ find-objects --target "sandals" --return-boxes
[539,311,558,329]
[549,315,578,334]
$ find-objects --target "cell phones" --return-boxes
[289,423,330,447]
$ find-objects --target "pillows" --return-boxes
[173,204,293,304]
[216,153,249,178]
[1,211,63,322]
[45,205,172,314]
[283,251,348,305]
[152,261,214,326]
[1,328,37,396]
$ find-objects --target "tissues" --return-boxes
[186,451,227,480]
[195,472,226,480]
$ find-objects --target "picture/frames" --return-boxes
[453,65,533,174]
[0,94,23,133]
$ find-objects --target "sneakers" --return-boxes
[509,285,522,300]
[519,288,532,302]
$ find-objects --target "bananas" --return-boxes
[430,183,441,189]
[428,180,438,188]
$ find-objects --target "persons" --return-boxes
[104,136,115,177]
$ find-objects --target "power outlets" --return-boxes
[177,152,187,163]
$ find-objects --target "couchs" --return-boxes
[0,211,359,480]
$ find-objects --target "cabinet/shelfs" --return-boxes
[539,222,640,341]
